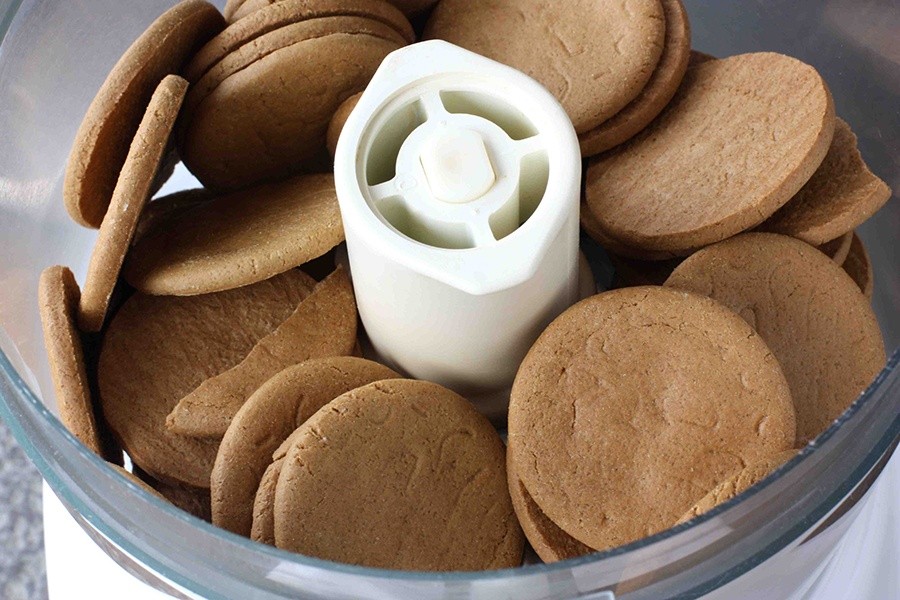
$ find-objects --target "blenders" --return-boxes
[0,0,899,600]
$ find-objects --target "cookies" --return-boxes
[36,0,891,571]
